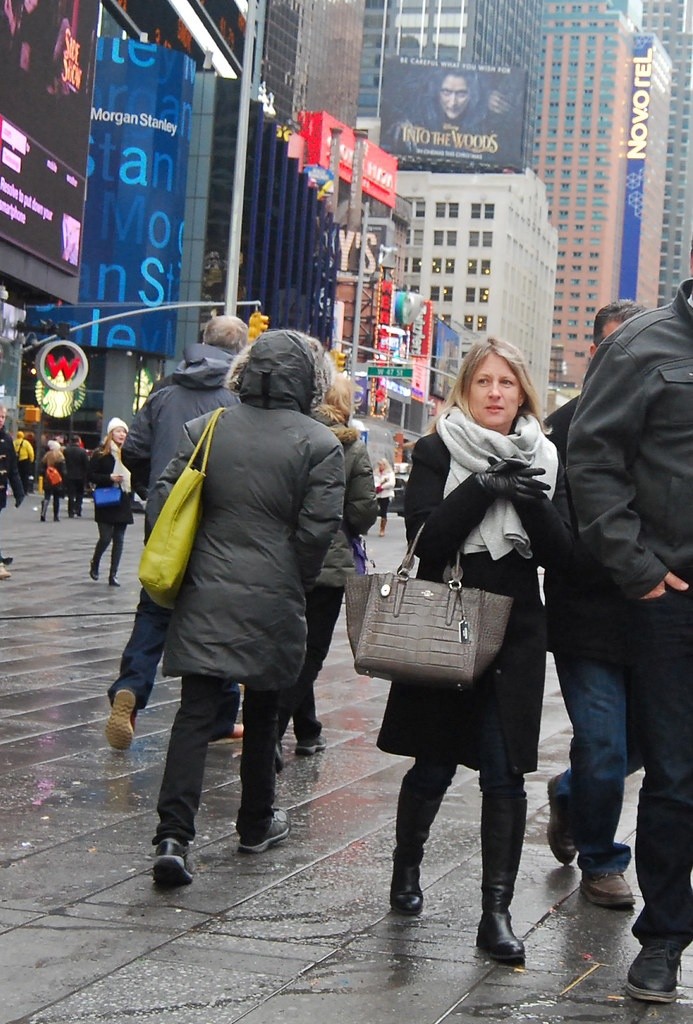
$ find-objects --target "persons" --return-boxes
[565,240,693,1004]
[0,404,24,580]
[13,431,34,495]
[63,435,90,518]
[376,336,575,960]
[388,67,510,153]
[38,439,66,522]
[145,329,346,885]
[105,315,249,750]
[88,417,135,587]
[373,457,396,537]
[274,375,377,771]
[543,299,651,908]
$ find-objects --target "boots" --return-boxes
[41,499,49,521]
[109,556,121,587]
[54,503,60,521]
[379,518,386,537]
[476,798,528,961]
[90,541,108,579]
[389,787,443,915]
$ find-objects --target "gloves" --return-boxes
[376,486,383,494]
[476,454,552,501]
[15,495,24,509]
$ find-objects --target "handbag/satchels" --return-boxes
[138,406,226,609]
[345,523,513,689]
[92,487,123,508]
[46,467,62,486]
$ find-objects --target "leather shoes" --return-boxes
[580,871,635,907]
[547,773,577,865]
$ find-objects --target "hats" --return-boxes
[107,417,129,436]
[47,440,62,450]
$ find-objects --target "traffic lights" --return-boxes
[248,311,270,345]
[331,348,347,375]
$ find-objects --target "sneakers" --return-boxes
[208,723,245,745]
[105,689,135,750]
[296,734,327,756]
[274,738,286,774]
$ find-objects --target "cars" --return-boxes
[377,478,405,516]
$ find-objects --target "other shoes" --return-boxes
[238,807,291,853]
[153,838,193,889]
[0,562,11,579]
[625,940,682,1003]
